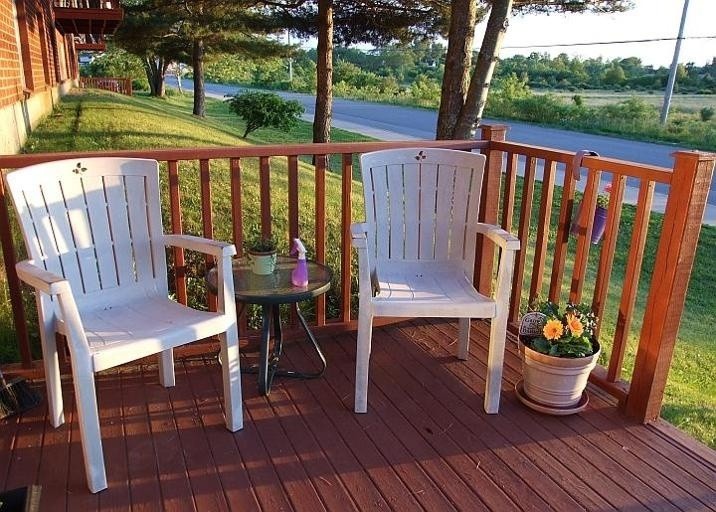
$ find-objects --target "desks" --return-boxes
[205,255,334,395]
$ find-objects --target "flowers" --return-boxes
[597,183,613,208]
[524,300,600,359]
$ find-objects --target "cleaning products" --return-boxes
[290,238,309,286]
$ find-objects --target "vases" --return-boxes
[570,200,608,245]
[514,333,602,416]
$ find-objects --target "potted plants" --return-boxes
[243,218,287,276]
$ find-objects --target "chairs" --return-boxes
[4,157,245,495]
[350,146,521,415]
